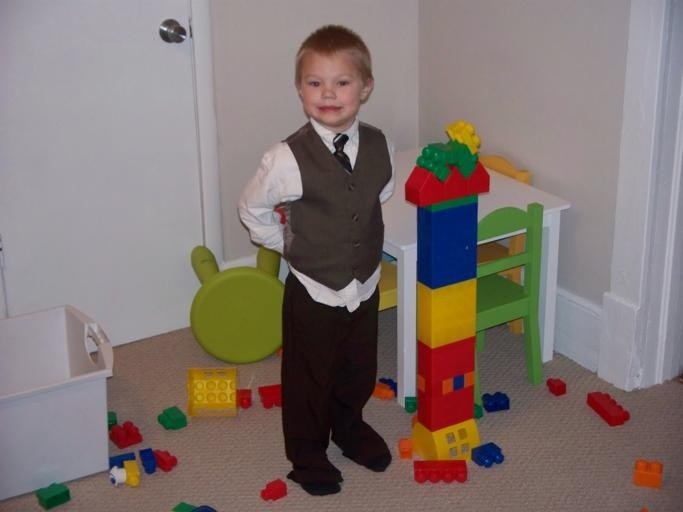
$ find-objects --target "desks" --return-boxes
[381,148,573,410]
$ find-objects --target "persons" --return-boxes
[234,23,396,498]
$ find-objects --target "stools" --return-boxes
[190,246,286,364]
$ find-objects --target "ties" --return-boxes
[332,134,352,171]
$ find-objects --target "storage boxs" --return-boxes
[0,304,114,502]
